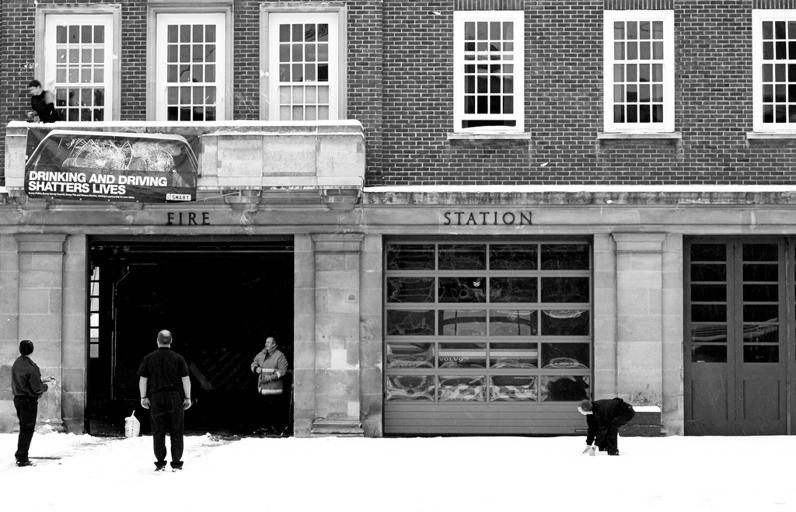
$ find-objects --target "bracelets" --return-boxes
[185,397,191,399]
[140,396,147,400]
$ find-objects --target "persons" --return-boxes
[11,339,57,467]
[24,80,60,123]
[136,328,194,470]
[250,336,289,435]
[577,397,636,457]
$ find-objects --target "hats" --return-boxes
[20,340,33,355]
